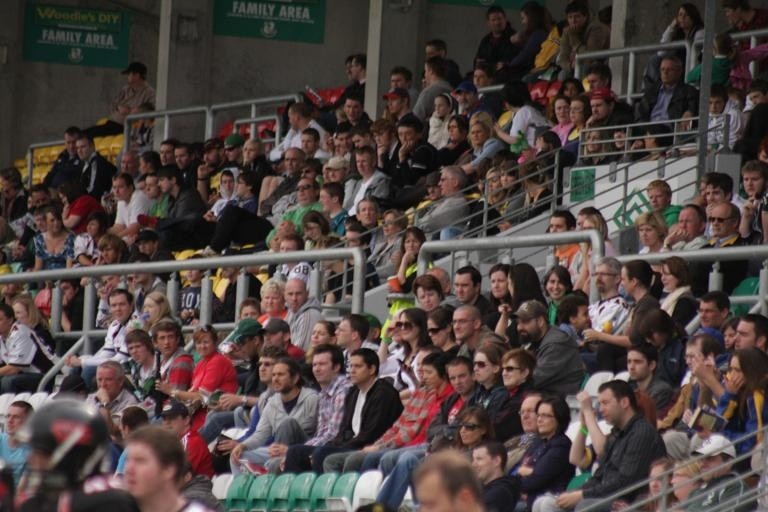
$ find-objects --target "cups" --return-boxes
[387,275,401,293]
[604,321,613,333]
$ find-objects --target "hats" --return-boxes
[226,318,265,344]
[131,80,617,241]
[688,433,739,462]
[155,401,192,421]
[120,61,149,79]
[256,317,292,338]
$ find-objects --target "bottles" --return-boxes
[304,84,327,107]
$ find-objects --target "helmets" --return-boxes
[9,398,110,478]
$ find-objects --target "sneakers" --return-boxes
[244,463,269,478]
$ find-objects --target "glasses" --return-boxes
[709,216,734,223]
[590,272,619,278]
[393,297,565,433]
[255,360,276,367]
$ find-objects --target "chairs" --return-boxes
[209,467,384,512]
[11,132,126,188]
